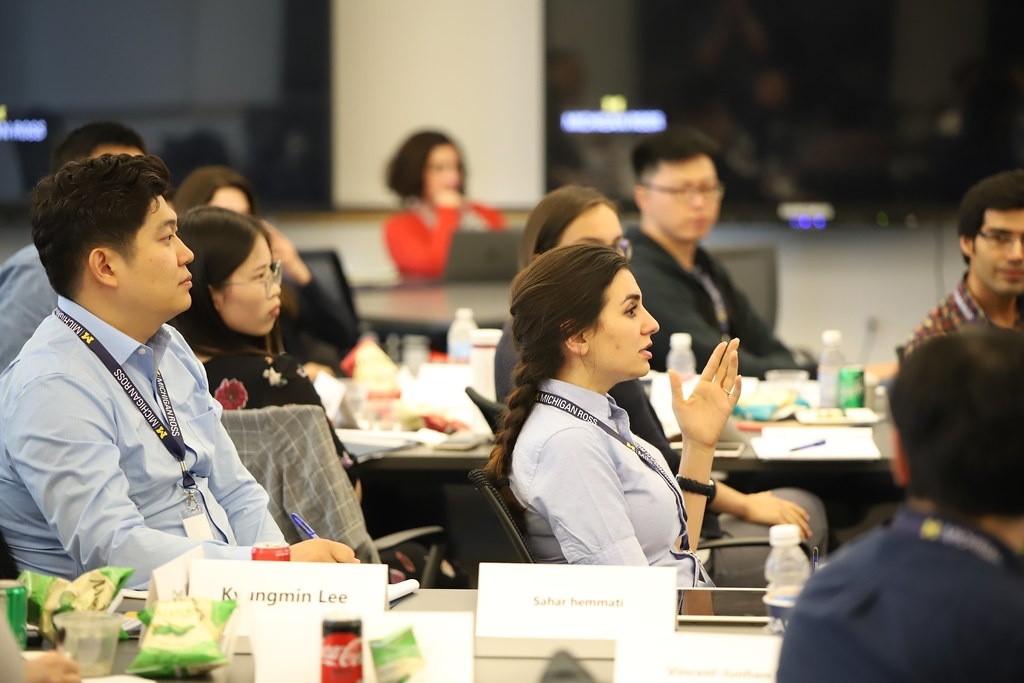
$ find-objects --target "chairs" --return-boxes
[220,226,778,586]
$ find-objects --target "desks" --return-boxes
[0,590,841,680]
[312,363,896,485]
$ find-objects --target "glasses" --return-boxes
[640,181,724,203]
[977,229,1024,248]
[610,238,634,260]
[223,258,284,295]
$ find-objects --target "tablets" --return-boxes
[676,586,771,627]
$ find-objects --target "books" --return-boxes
[387,578,421,602]
[434,430,491,450]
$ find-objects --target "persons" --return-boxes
[483,244,741,605]
[775,325,1023,683]
[902,174,1023,354]
[381,130,508,280]
[621,128,817,378]
[0,120,466,589]
[494,184,830,586]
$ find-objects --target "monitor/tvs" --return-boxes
[540,0,1023,226]
[0,0,334,213]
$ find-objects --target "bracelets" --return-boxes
[675,472,717,504]
[252,546,290,560]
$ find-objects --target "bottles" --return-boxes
[666,333,696,381]
[818,330,843,409]
[764,525,811,601]
[447,309,480,363]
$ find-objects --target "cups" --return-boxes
[52,610,123,677]
[762,595,810,627]
[764,369,810,386]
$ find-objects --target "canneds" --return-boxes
[0,580,28,652]
[252,542,291,561]
[837,368,865,409]
[320,616,363,683]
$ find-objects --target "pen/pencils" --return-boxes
[291,513,319,539]
[737,426,761,432]
[791,439,826,452]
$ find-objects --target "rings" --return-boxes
[723,387,731,396]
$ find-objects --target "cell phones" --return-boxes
[437,438,482,451]
